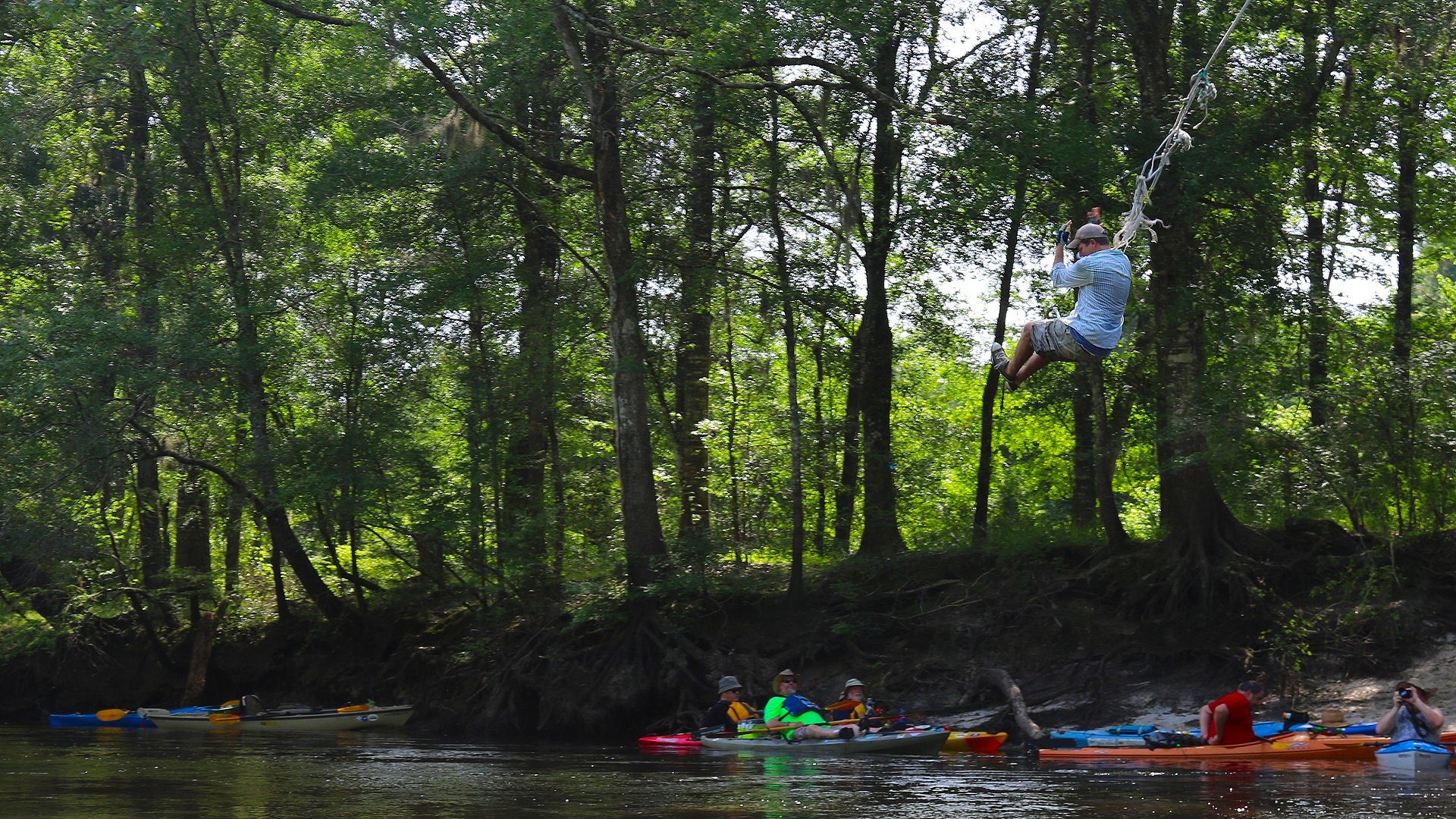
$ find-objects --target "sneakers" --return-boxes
[991,342,1016,383]
[1005,380,1021,393]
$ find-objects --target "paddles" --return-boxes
[209,704,368,723]
[1321,742,1456,748]
[1254,735,1293,750]
[96,699,239,722]
[692,711,925,738]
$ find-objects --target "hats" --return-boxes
[837,678,868,702]
[1395,678,1433,699]
[718,676,744,695]
[778,669,802,677]
[1066,222,1108,249]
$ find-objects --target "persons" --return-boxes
[990,206,1132,391]
[703,676,761,739]
[764,668,867,741]
[833,679,907,732]
[1376,677,1444,744]
[1199,680,1266,746]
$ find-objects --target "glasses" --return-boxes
[727,688,742,694]
[779,679,799,683]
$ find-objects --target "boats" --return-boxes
[1047,721,1381,748]
[640,718,950,757]
[1040,728,1456,760]
[1375,738,1452,772]
[939,728,1007,754]
[48,705,236,730]
[137,687,416,734]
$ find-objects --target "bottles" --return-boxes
[899,707,906,723]
[1283,712,1292,719]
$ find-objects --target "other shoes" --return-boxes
[877,721,907,737]
[838,727,854,740]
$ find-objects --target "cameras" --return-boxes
[1398,687,1411,699]
